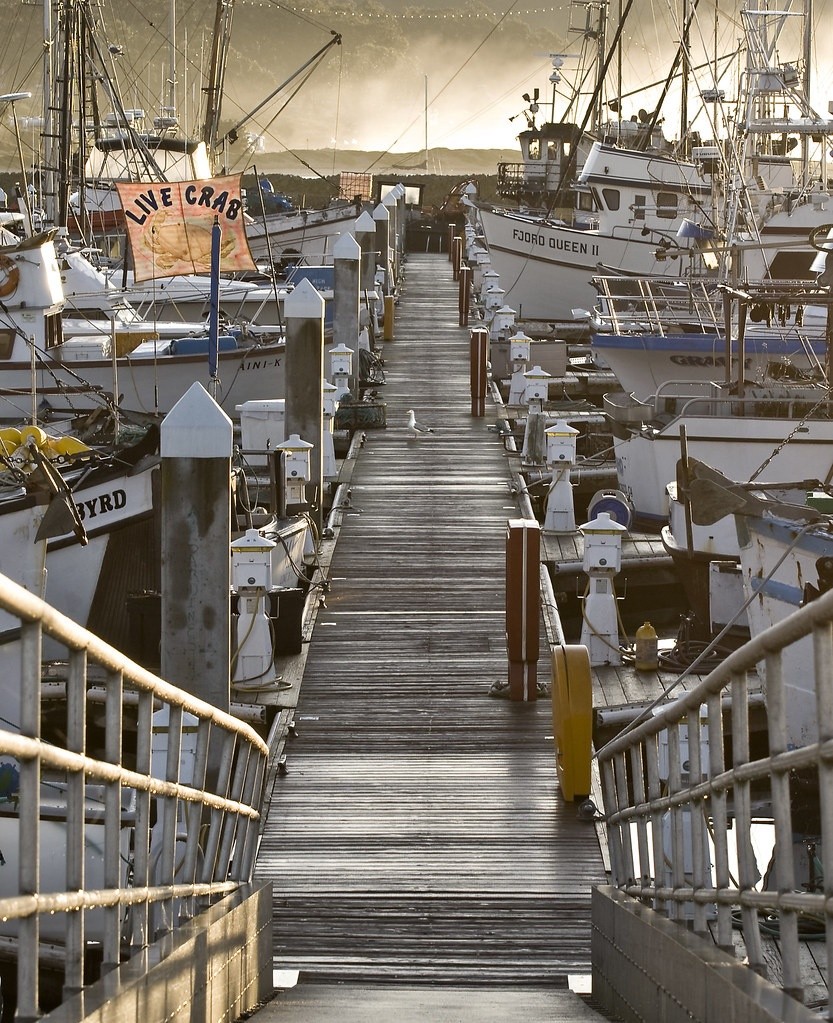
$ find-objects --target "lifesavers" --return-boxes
[0,254,20,297]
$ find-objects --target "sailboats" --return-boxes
[464,1,833,813]
[1,0,365,972]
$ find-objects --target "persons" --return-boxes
[11,182,22,211]
[518,201,530,216]
[258,177,279,215]
[27,180,38,208]
[536,202,549,218]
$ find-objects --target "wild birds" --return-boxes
[405,409,435,439]
